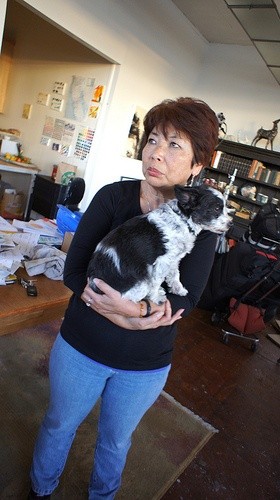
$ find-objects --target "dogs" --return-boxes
[87,183,236,306]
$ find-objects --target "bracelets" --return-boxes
[143,297,152,319]
[137,300,145,319]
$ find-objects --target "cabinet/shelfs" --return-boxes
[25,174,69,222]
[0,39,16,113]
[191,138,280,269]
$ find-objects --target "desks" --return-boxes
[0,159,41,222]
[0,217,77,339]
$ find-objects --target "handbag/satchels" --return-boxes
[196,242,280,314]
[240,203,280,252]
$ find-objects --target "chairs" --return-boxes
[62,176,85,213]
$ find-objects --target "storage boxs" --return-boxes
[0,180,27,220]
[55,202,83,252]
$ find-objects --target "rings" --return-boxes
[85,298,93,307]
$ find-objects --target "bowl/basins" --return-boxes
[55,204,83,235]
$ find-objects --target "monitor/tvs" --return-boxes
[0,165,34,224]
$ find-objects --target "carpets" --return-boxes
[0,315,219,500]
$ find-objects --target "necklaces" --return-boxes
[145,180,152,213]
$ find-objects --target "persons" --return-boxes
[24,95,221,500]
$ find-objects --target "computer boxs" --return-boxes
[31,174,69,220]
